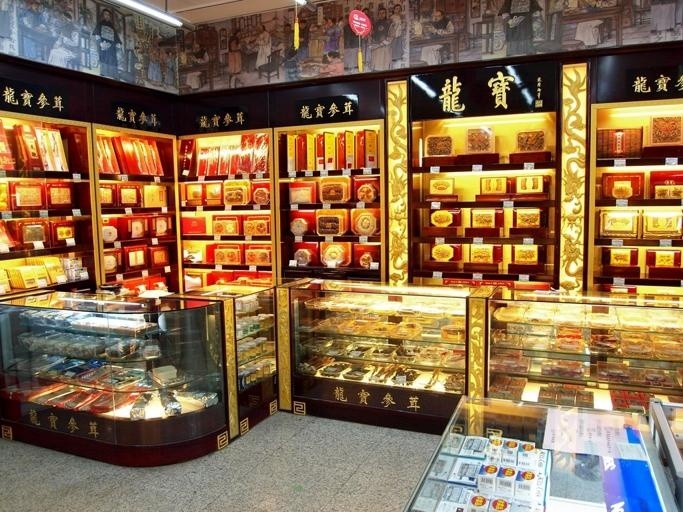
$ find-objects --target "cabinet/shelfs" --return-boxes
[2,290,231,468]
[2,55,101,293]
[92,74,183,292]
[406,397,682,512]
[180,82,277,284]
[276,277,497,437]
[586,40,683,296]
[164,283,278,442]
[488,285,683,410]
[384,50,589,289]
[270,72,388,281]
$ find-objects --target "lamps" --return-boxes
[108,0,198,33]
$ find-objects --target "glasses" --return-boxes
[108,0,198,33]
